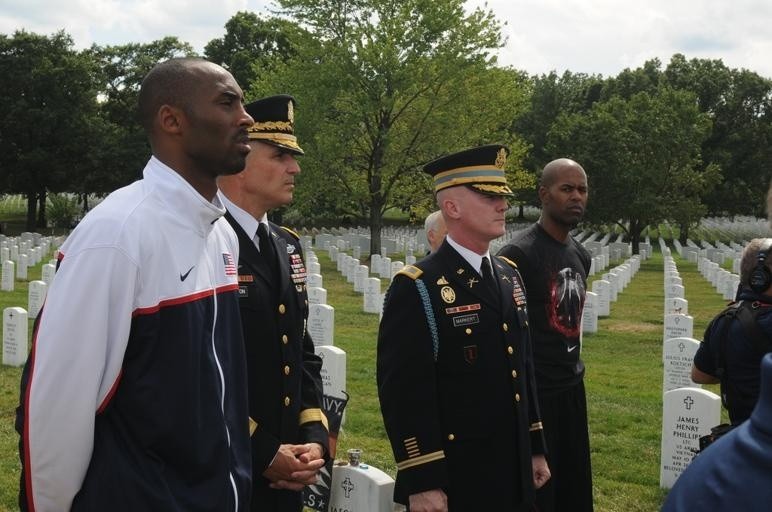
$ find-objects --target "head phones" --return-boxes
[748,238,772,293]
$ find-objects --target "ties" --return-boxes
[480,256,499,297]
[256,222,278,272]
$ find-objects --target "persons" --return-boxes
[492,157,595,511]
[425,208,448,256]
[375,143,554,511]
[216,94,333,510]
[688,237,772,429]
[13,57,257,511]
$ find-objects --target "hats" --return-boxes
[422,144,515,197]
[242,95,305,156]
